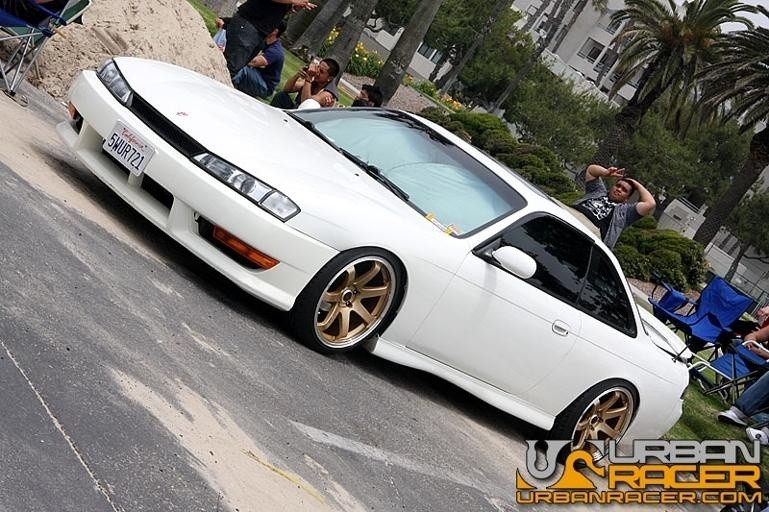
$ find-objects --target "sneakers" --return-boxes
[718,411,747,427]
[746,427,769,446]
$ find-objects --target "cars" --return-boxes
[57,56,695,472]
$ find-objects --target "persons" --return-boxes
[568,164,656,252]
[718,325,769,446]
[215,0,383,109]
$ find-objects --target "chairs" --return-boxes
[644,274,768,408]
[1,0,96,108]
